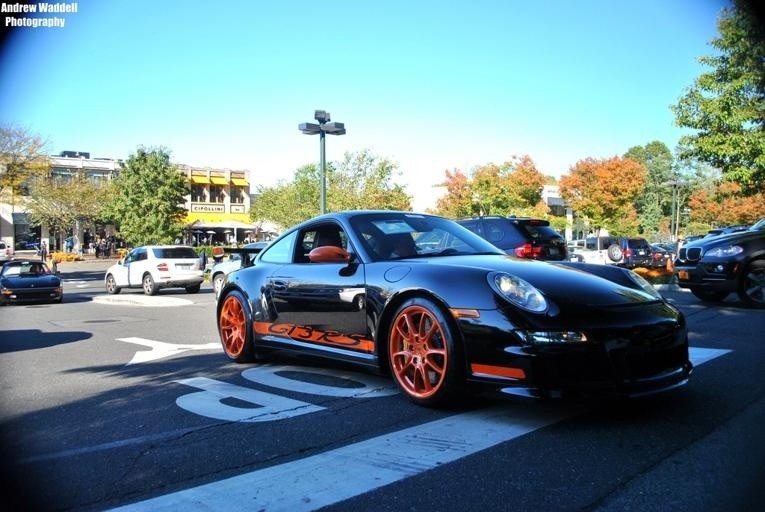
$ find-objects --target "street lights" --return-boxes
[297,106,350,217]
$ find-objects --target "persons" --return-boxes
[23,226,263,262]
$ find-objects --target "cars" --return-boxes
[210,241,271,298]
[649,235,702,268]
[0,261,63,304]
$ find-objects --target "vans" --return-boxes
[0,242,11,262]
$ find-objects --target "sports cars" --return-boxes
[210,205,695,416]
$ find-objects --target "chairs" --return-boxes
[309,226,350,262]
[374,236,395,258]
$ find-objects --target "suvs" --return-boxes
[415,213,571,265]
[672,218,764,308]
[703,224,751,239]
[615,237,653,267]
[576,236,625,267]
[104,245,205,296]
